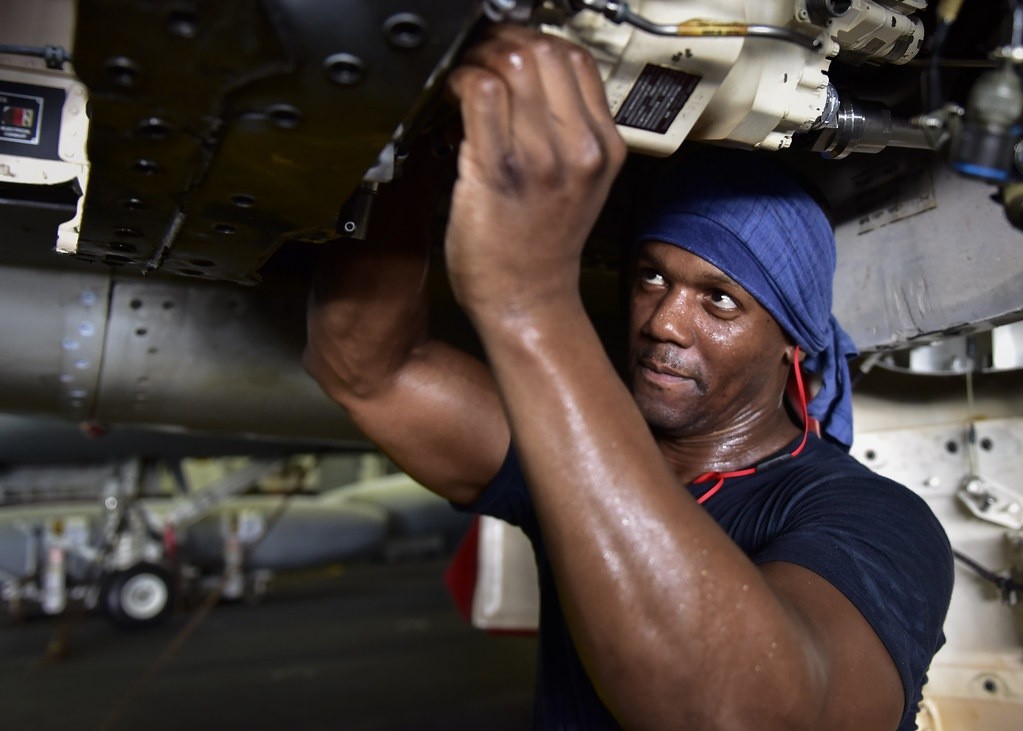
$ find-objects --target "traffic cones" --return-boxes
[28,548,72,624]
[214,533,257,604]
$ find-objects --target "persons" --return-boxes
[299,27,955,731]
